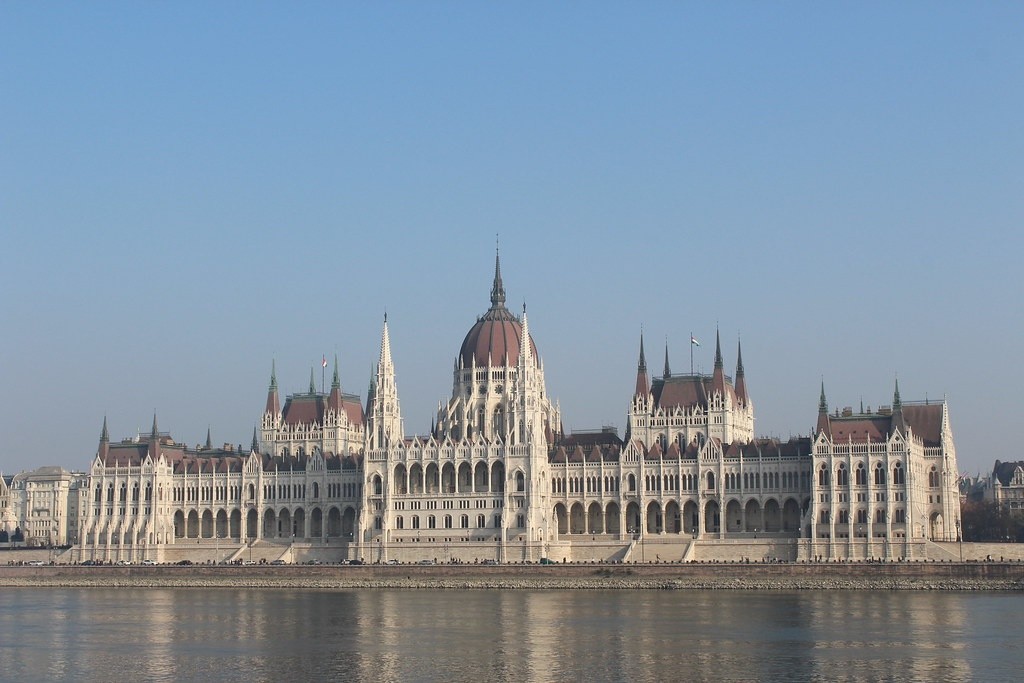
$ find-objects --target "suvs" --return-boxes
[143,559,156,566]
[272,560,285,566]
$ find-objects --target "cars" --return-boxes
[309,559,319,565]
[352,558,362,565]
[81,561,95,566]
[245,560,256,565]
[419,560,432,565]
[341,559,351,565]
[117,561,130,566]
[178,560,192,565]
[540,558,556,565]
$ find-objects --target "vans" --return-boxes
[486,559,498,565]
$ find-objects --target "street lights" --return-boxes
[545,543,549,566]
[630,526,635,564]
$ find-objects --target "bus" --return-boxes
[30,560,44,566]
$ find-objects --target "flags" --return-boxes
[692,337,699,346]
[322,359,327,367]
